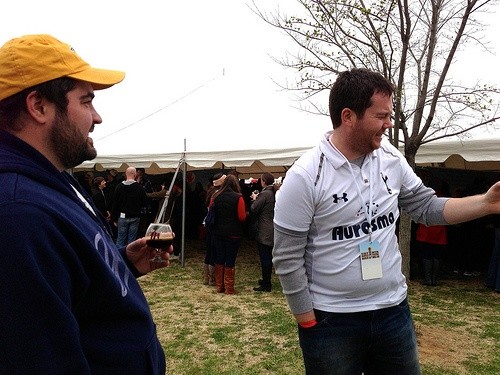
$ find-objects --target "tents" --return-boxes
[65,75,500,268]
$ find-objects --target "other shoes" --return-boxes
[494,288,500,293]
[253,286,271,292]
[485,285,495,291]
[258,278,263,284]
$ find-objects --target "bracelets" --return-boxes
[299,319,318,328]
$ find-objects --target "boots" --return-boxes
[420,258,432,285]
[203,263,209,285]
[208,264,216,286]
[224,267,240,295]
[432,259,441,285]
[215,264,224,293]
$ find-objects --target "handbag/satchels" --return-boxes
[198,225,208,240]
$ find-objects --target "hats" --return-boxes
[0,34,126,101]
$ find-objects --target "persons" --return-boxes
[416,180,500,293]
[0,35,166,375]
[81,166,183,260]
[201,172,281,295]
[273,68,500,375]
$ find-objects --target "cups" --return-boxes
[254,190,258,196]
[144,224,173,262]
